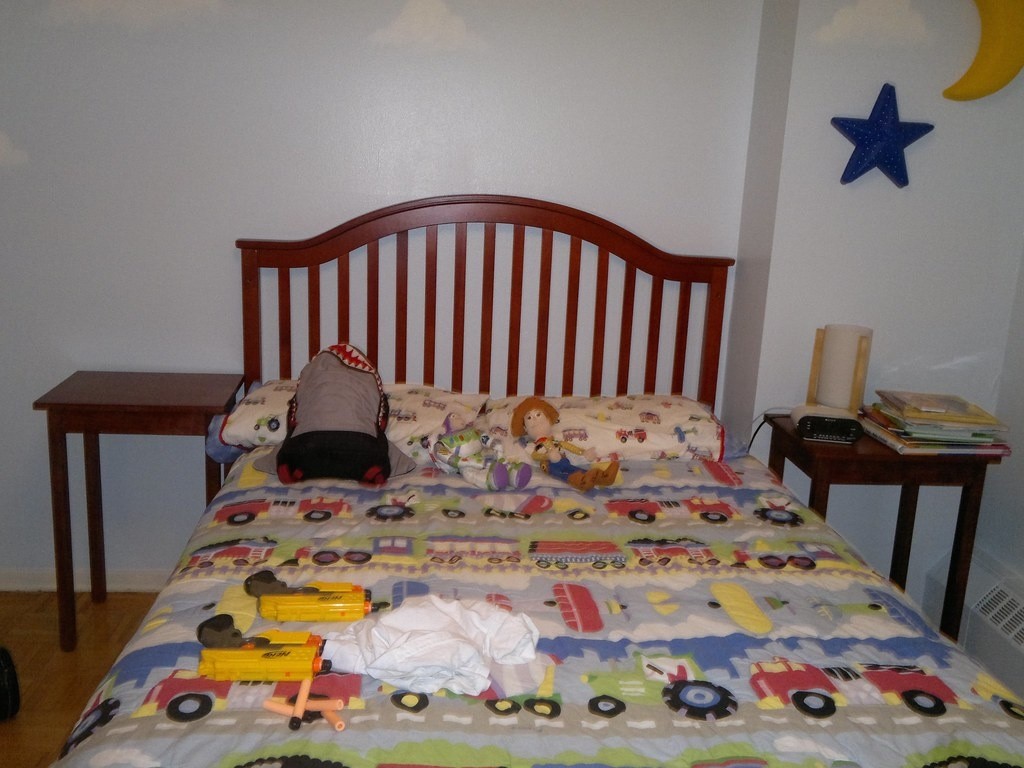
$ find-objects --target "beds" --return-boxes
[53,194,1024,768]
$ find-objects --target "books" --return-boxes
[860,390,1012,456]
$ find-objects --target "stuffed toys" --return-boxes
[433,413,531,491]
[511,396,619,491]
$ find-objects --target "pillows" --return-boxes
[486,396,747,466]
[207,379,488,464]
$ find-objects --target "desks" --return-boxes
[31,370,242,652]
[762,413,1002,644]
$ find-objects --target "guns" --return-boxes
[239,566,396,624]
[194,612,338,684]
